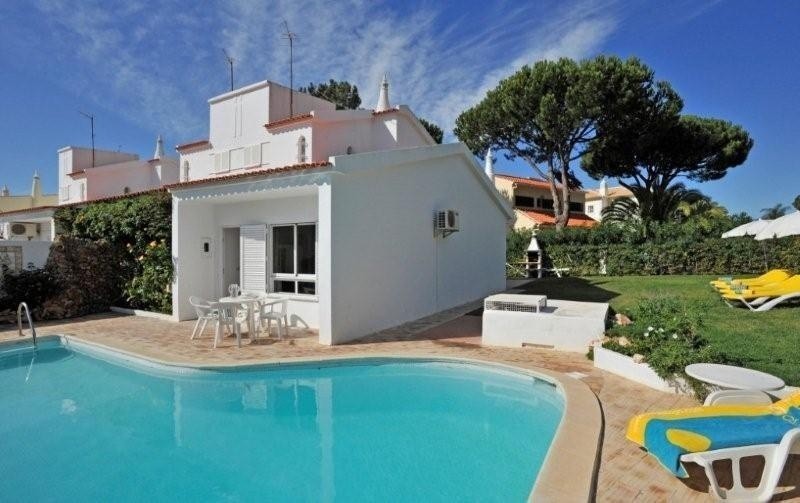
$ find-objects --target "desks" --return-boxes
[684,363,784,389]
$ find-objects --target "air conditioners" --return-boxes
[3,222,37,238]
[435,208,463,232]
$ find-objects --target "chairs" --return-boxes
[709,269,800,313]
[629,389,800,502]
[189,291,292,348]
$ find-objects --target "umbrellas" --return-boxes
[721,211,800,272]
